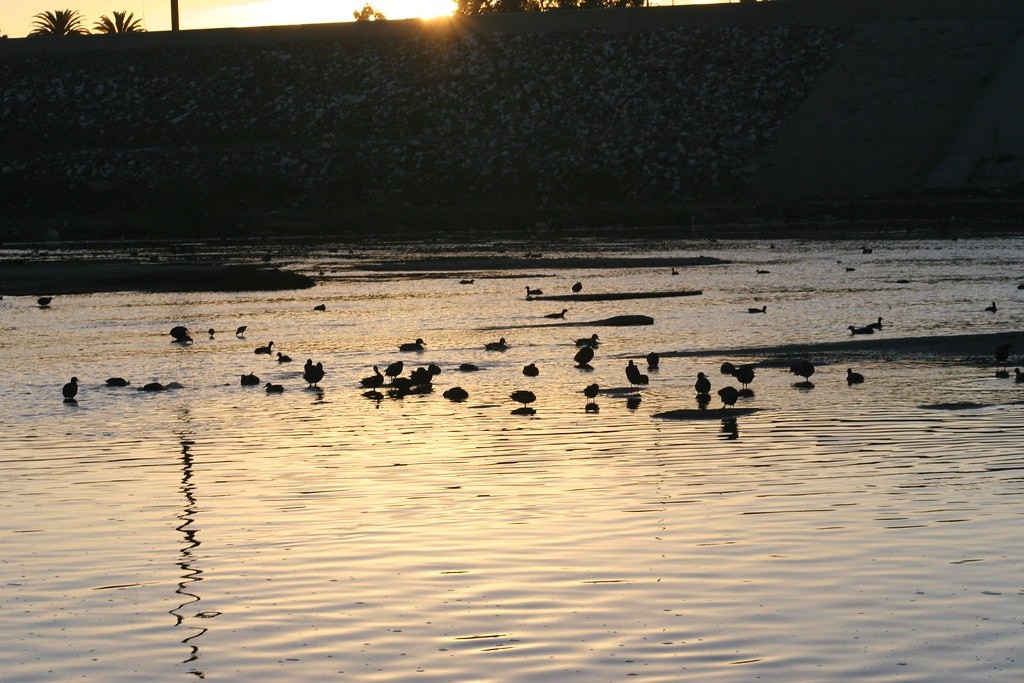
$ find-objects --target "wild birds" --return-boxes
[138,382,168,390]
[170,304,326,362]
[262,382,284,392]
[510,390,536,407]
[241,374,260,385]
[303,359,325,387]
[359,246,883,389]
[695,372,711,395]
[732,365,755,389]
[647,352,660,369]
[62,377,80,399]
[625,360,642,388]
[720,362,735,374]
[105,377,131,385]
[37,296,53,306]
[789,360,815,382]
[584,384,600,403]
[442,387,469,397]
[718,386,739,408]
